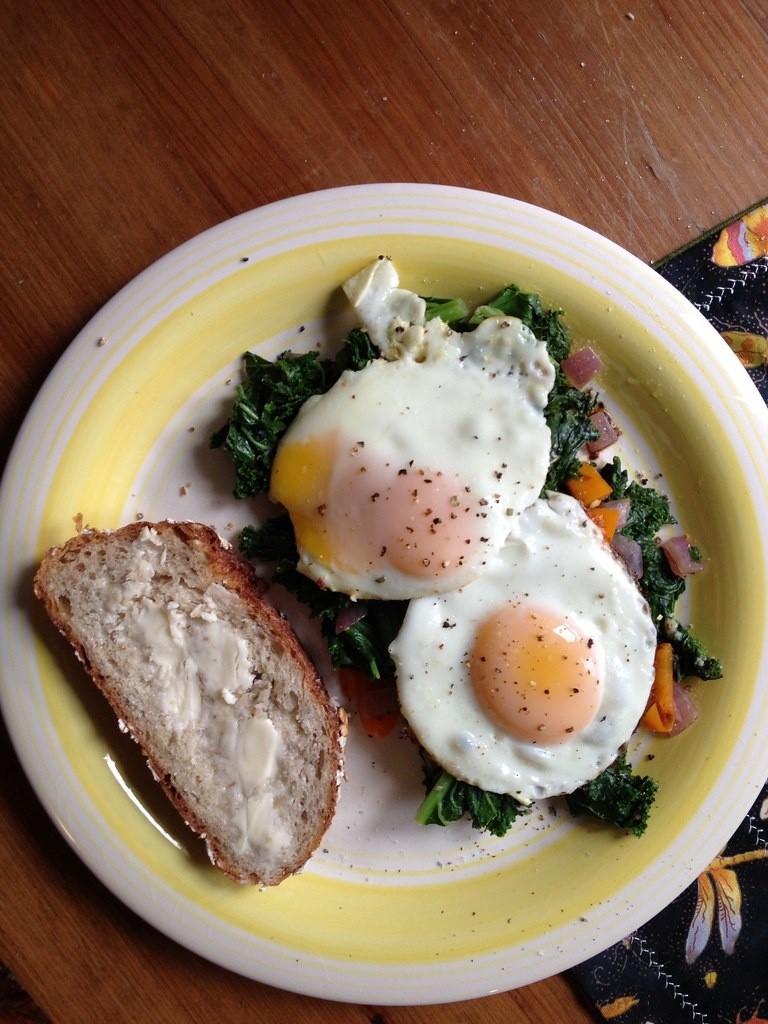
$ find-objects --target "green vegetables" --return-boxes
[206,284,725,843]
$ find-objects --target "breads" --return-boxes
[31,518,344,886]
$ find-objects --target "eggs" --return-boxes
[268,260,558,602]
[388,490,658,806]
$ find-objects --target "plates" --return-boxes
[1,182,768,1007]
